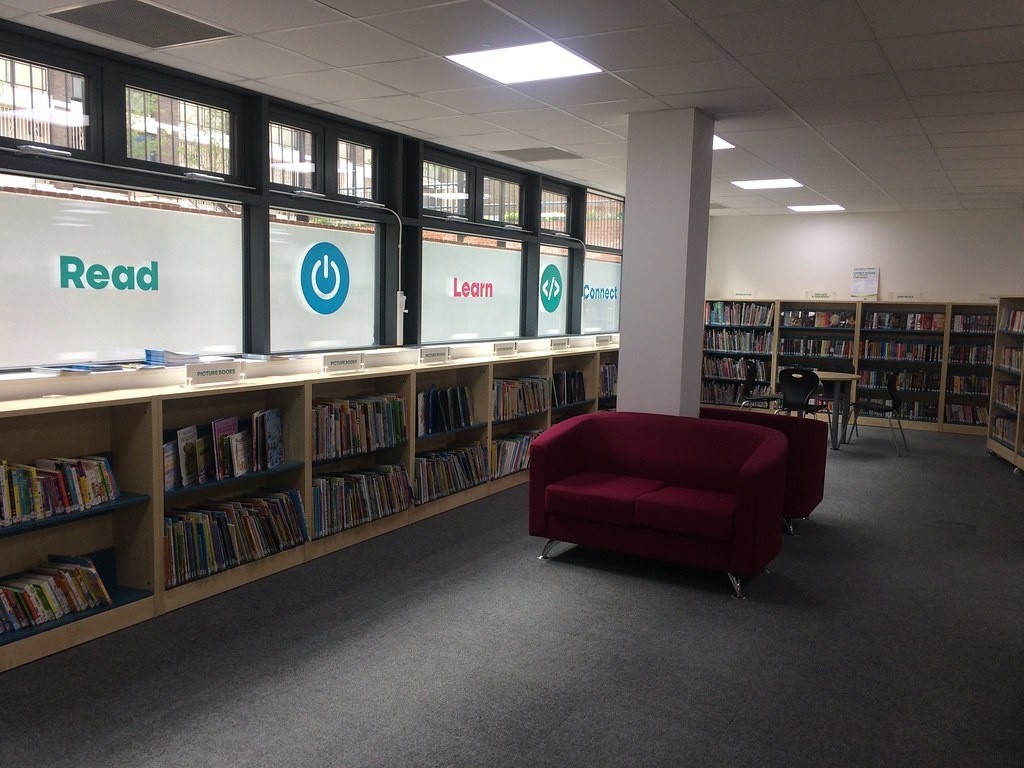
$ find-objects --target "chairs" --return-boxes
[835,368,910,457]
[812,360,860,439]
[740,359,784,416]
[772,366,832,436]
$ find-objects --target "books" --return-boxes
[31,367,91,375]
[0,555,113,636]
[492,376,552,422]
[417,385,474,437]
[199,356,234,363]
[164,486,311,595]
[992,306,1024,451]
[600,360,618,397]
[312,393,408,462]
[72,364,121,372]
[242,354,294,361]
[415,440,488,505]
[699,301,997,427]
[491,429,545,481]
[163,407,283,493]
[144,349,199,367]
[552,369,586,407]
[312,464,415,539]
[122,363,165,370]
[0,456,119,526]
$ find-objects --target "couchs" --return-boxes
[699,407,829,538]
[527,411,790,599]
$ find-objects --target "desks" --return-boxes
[791,370,862,449]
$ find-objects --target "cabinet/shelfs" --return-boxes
[702,297,999,434]
[983,295,1024,476]
[0,343,621,675]
